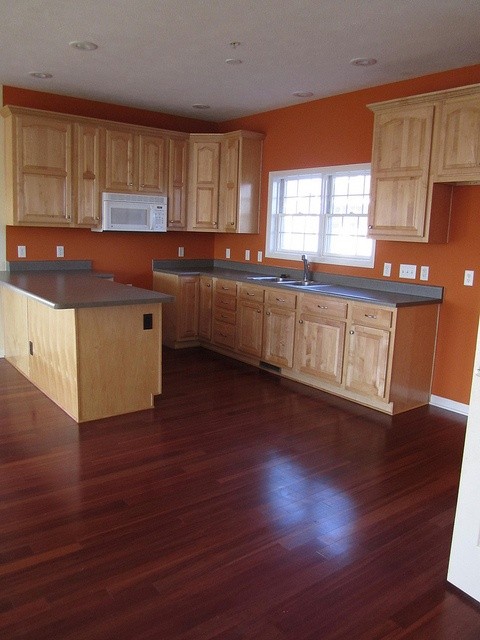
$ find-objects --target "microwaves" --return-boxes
[101,192,168,234]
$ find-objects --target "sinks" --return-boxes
[249,276,302,282]
[281,281,333,286]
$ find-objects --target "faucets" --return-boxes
[302,255,311,281]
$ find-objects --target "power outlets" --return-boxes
[398,263,417,279]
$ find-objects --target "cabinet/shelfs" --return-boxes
[101,119,168,196]
[297,292,348,400]
[200,275,214,351]
[190,134,224,233]
[237,281,297,382]
[153,270,200,349]
[364,88,455,246]
[75,115,101,229]
[168,130,190,231]
[0,104,75,228]
[345,299,441,416]
[433,84,480,185]
[224,129,265,234]
[212,277,237,360]
